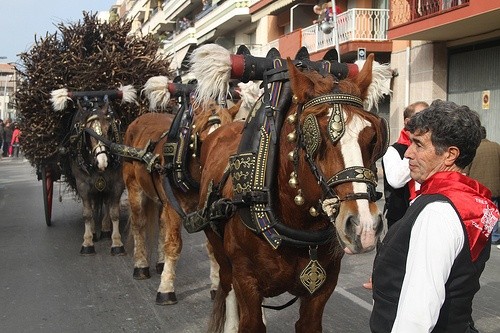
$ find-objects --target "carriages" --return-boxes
[37,43,394,333]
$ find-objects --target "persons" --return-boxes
[313,2,346,48]
[371,101,500,333]
[381,101,428,229]
[463,126,500,243]
[179,17,190,32]
[202,0,209,11]
[0,119,22,160]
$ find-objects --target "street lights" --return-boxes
[321,0,340,63]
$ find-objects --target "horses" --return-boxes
[177,40,393,333]
[47,81,138,256]
[124,71,277,333]
